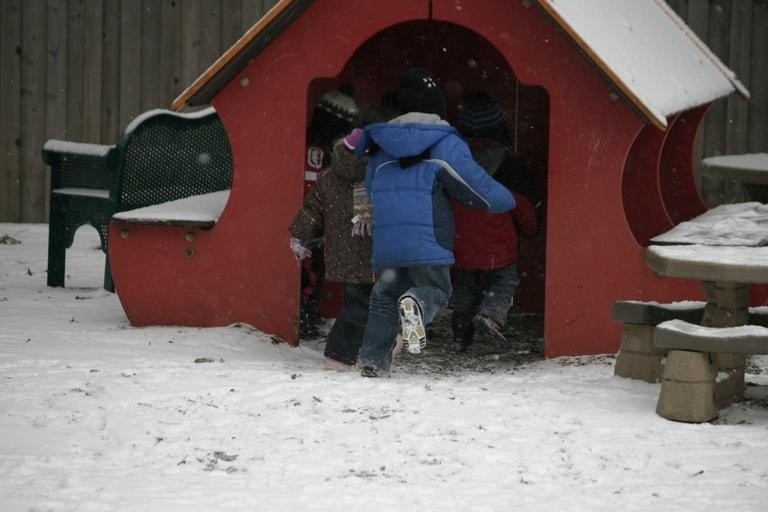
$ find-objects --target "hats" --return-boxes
[456,90,506,137]
[312,82,359,143]
[393,69,447,119]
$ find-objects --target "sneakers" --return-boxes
[362,365,391,376]
[452,329,468,353]
[399,297,427,356]
[323,358,350,372]
[472,314,511,351]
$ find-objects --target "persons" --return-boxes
[287,69,539,377]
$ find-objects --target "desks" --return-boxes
[644,200,768,412]
[700,152,767,205]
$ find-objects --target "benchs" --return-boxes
[611,299,768,384]
[653,318,768,423]
[41,105,235,295]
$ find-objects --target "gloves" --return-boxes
[289,238,312,263]
